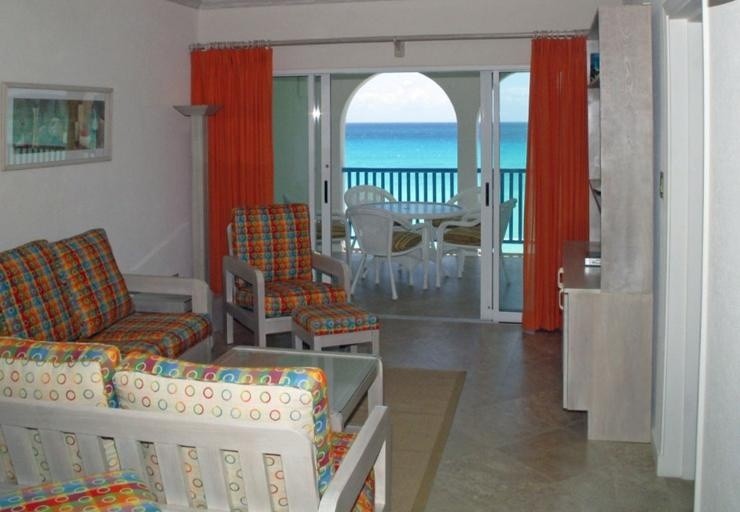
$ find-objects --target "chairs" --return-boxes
[222,202,356,348]
[339,182,517,301]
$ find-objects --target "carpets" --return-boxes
[204,342,467,512]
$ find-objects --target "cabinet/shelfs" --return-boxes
[556,0,654,444]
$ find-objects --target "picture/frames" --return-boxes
[0,82,114,173]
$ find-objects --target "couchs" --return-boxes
[1,335,397,512]
[0,227,216,356]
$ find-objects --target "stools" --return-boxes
[289,303,381,354]
